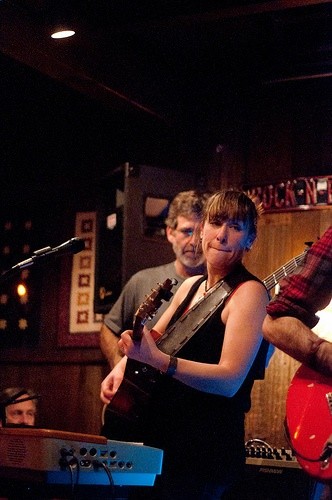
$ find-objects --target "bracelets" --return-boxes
[307,339,326,367]
[160,355,178,379]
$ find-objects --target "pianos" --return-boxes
[0,422,164,487]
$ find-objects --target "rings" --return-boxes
[120,347,124,352]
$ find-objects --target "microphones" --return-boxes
[11,237,85,271]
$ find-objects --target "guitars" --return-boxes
[259,241,316,292]
[102,278,179,439]
[285,363,332,479]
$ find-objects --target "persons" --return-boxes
[99,190,211,369]
[262,224,332,500]
[101,190,270,500]
[3,387,37,426]
